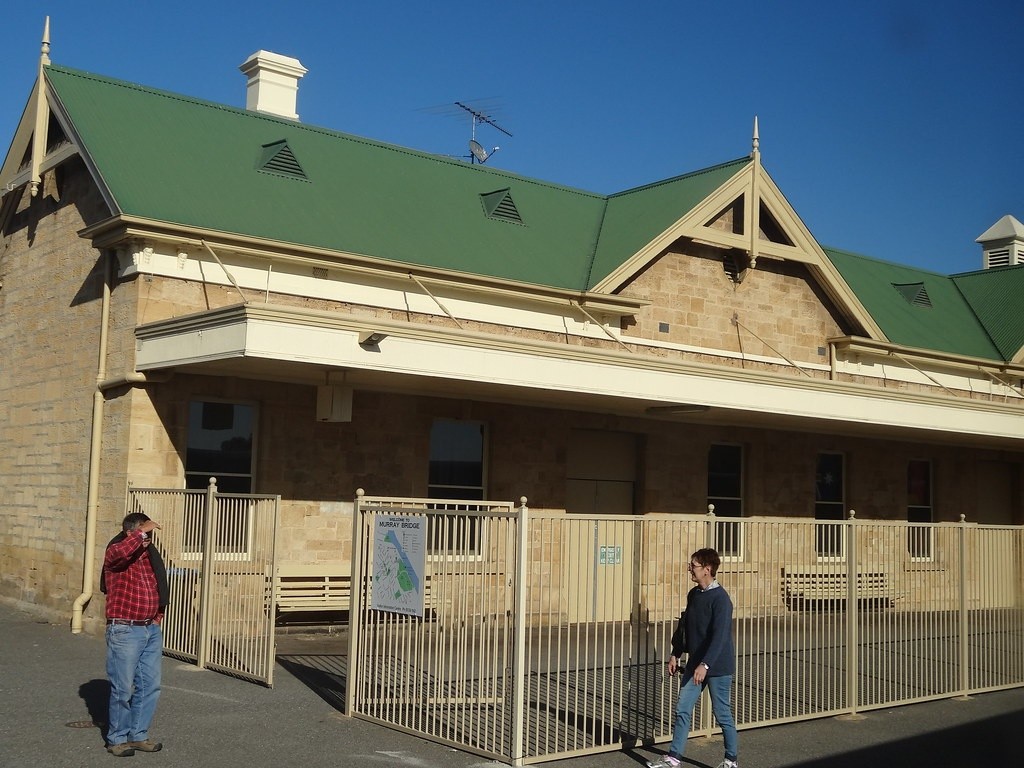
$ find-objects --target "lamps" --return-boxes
[358,330,389,344]
[646,406,710,416]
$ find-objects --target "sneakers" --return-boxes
[716,758,738,768]
[107,740,163,757]
[646,755,682,768]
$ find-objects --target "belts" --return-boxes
[106,619,152,626]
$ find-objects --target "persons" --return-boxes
[647,550,740,768]
[100,514,169,757]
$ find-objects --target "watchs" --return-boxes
[701,662,709,670]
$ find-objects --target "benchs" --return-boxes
[784,564,906,613]
[267,563,437,625]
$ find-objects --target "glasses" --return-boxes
[688,563,703,571]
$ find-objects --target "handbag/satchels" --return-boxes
[677,652,690,674]
